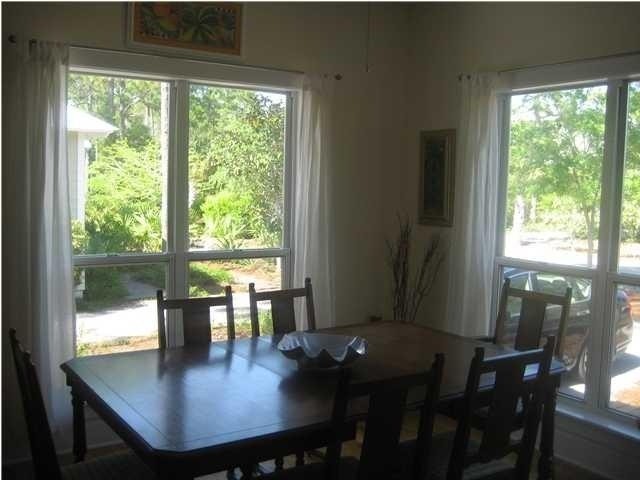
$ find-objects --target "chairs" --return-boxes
[250,277,344,467]
[154,289,280,478]
[256,353,448,479]
[399,339,553,480]
[9,333,151,478]
[425,278,571,460]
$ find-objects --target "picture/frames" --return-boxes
[125,1,247,61]
[417,127,458,227]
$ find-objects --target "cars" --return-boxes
[476,267,632,385]
